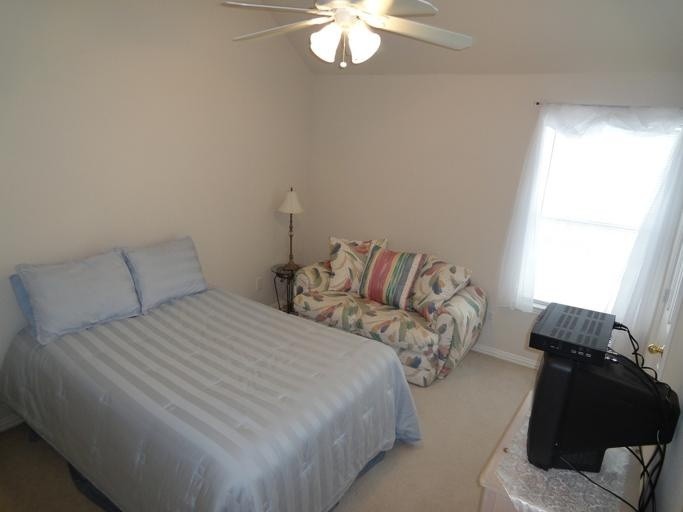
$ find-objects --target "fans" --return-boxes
[221,0,472,52]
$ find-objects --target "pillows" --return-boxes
[10,275,36,329]
[124,237,209,315]
[358,242,430,313]
[406,256,472,321]
[329,235,390,291]
[17,249,141,346]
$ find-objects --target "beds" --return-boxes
[1,288,423,512]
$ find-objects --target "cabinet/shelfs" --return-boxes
[479,387,641,512]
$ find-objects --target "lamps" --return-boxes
[278,188,304,270]
[310,1,392,66]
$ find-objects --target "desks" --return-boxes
[271,263,301,313]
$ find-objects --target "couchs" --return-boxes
[293,257,487,387]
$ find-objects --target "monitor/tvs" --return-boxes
[526,350,679,472]
